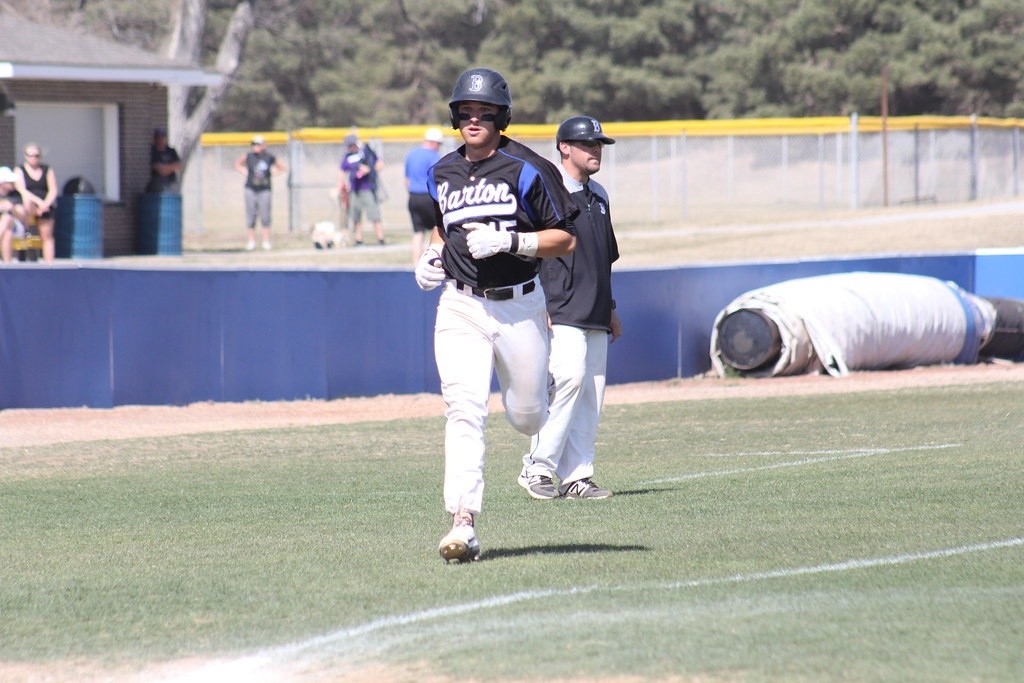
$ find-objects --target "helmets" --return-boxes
[556,116,615,150]
[448,67,512,131]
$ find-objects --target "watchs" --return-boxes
[611,300,617,310]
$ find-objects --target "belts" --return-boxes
[457,279,535,301]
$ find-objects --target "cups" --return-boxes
[29,249,37,261]
[19,250,25,260]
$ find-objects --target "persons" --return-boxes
[0,142,59,262]
[406,127,444,271]
[144,127,182,191]
[332,137,385,245]
[234,135,285,250]
[516,115,621,500]
[416,69,580,560]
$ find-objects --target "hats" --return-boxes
[0,167,19,183]
[154,126,166,137]
[344,135,357,146]
[427,130,444,143]
[251,137,264,145]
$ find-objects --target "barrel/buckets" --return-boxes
[103,206,136,255]
[135,194,182,256]
[55,195,102,260]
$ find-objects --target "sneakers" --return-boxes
[518,474,559,499]
[439,524,479,562]
[561,478,612,499]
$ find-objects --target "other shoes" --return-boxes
[261,240,270,251]
[246,240,256,251]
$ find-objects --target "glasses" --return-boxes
[24,151,41,158]
[581,139,603,148]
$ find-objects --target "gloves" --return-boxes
[416,249,446,291]
[462,222,513,259]
